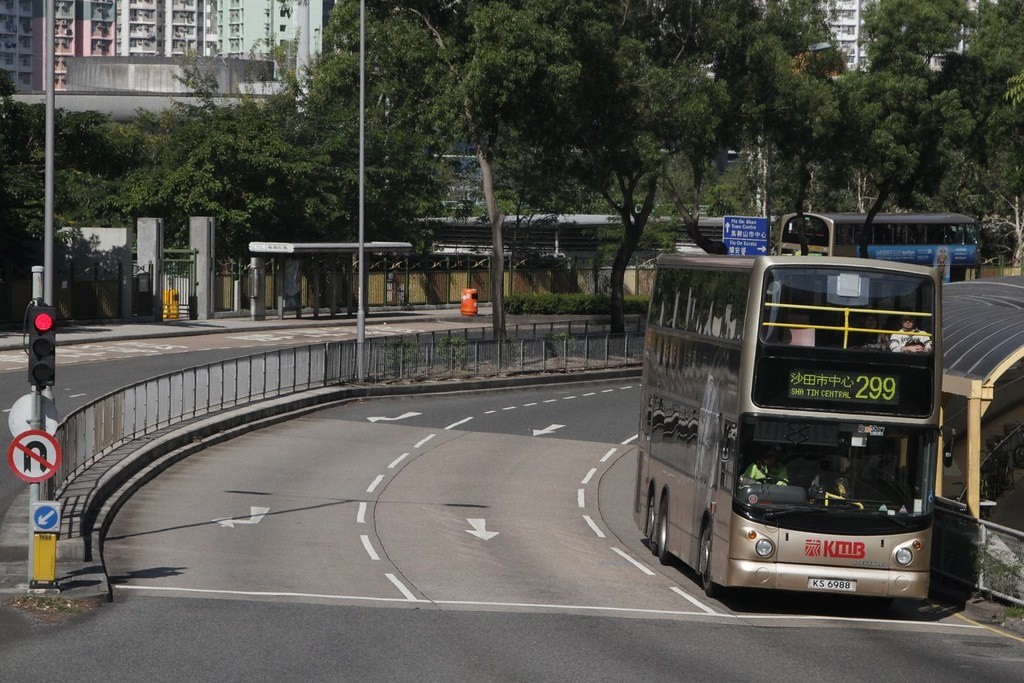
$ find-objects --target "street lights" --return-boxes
[727,150,767,218]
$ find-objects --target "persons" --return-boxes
[739,448,788,486]
[889,308,933,354]
[849,312,888,351]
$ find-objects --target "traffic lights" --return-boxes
[28,305,56,389]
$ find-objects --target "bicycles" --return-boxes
[971,421,1024,518]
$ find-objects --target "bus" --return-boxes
[778,210,981,290]
[631,250,957,609]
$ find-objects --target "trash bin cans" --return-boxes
[164,290,179,319]
[461,289,478,317]
